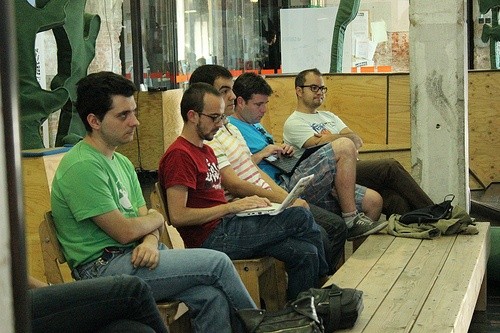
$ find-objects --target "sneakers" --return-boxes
[347,211,389,241]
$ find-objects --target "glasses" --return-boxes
[258,127,274,144]
[302,84,328,95]
[198,111,227,125]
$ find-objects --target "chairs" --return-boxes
[151,181,286,312]
[39,211,179,325]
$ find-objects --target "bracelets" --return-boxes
[149,232,160,242]
[47,282,52,287]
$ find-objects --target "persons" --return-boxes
[189,65,349,275]
[158,81,332,301]
[225,72,388,240]
[27,272,170,333]
[283,68,436,215]
[51,71,260,333]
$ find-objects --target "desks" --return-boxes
[320,222,490,333]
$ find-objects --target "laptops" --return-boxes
[236,174,314,218]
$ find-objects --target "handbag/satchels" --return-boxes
[238,295,324,333]
[291,284,364,329]
[400,194,455,226]
